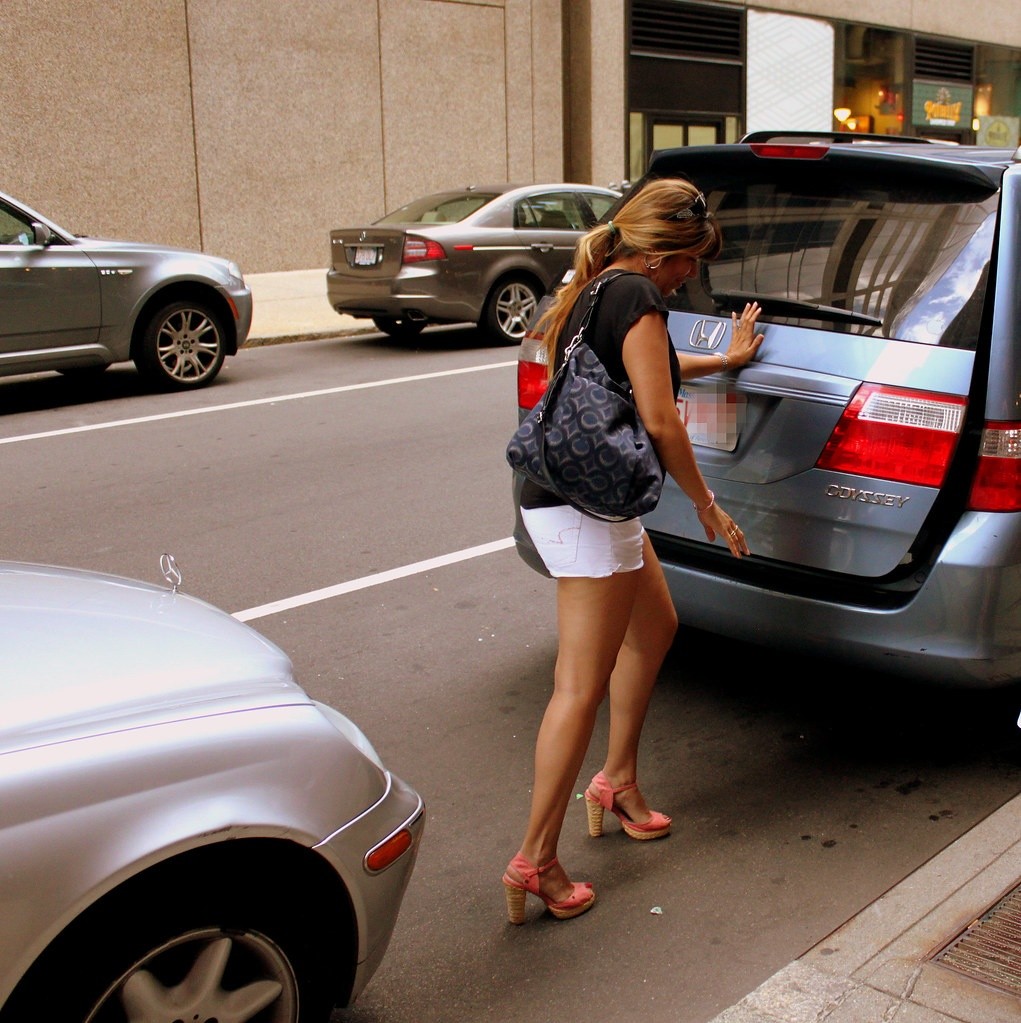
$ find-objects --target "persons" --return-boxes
[504,178,764,924]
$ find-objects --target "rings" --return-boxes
[737,325,742,328]
[735,525,738,532]
[731,530,736,537]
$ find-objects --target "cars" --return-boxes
[326,182,622,345]
[1,191,254,394]
[0,555,425,1023]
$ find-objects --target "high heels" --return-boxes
[583,769,672,839]
[503,851,598,924]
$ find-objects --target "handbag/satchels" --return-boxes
[505,271,675,522]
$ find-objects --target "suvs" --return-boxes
[508,129,1021,725]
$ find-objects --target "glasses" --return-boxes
[658,192,709,225]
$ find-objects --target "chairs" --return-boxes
[541,210,572,228]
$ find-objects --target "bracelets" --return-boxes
[693,490,714,513]
[713,352,728,372]
